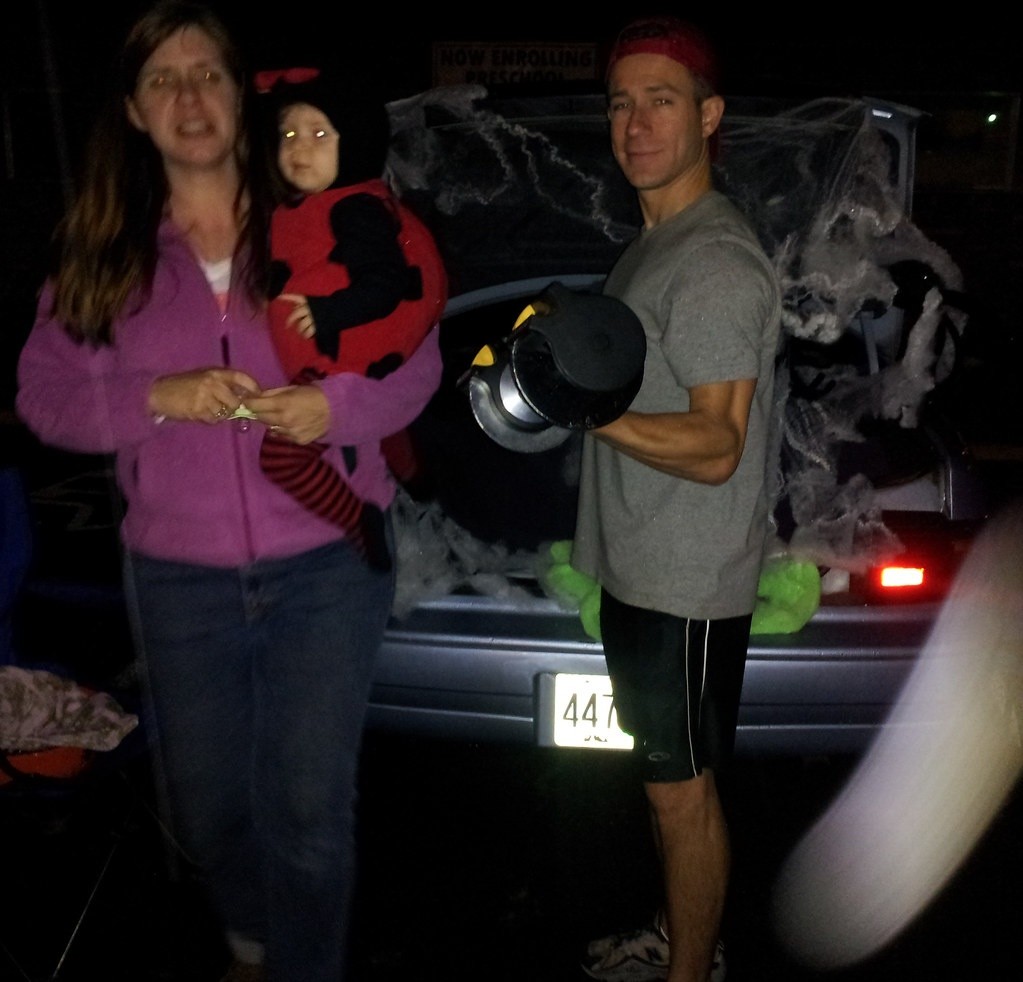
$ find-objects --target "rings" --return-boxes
[214,403,226,418]
[269,424,279,435]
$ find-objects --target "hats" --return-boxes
[604,15,722,163]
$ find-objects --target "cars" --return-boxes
[238,36,994,768]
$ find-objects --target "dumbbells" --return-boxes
[469,290,649,455]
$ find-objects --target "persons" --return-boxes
[569,15,785,978]
[259,72,438,575]
[19,0,398,980]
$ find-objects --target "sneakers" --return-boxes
[582,910,724,980]
